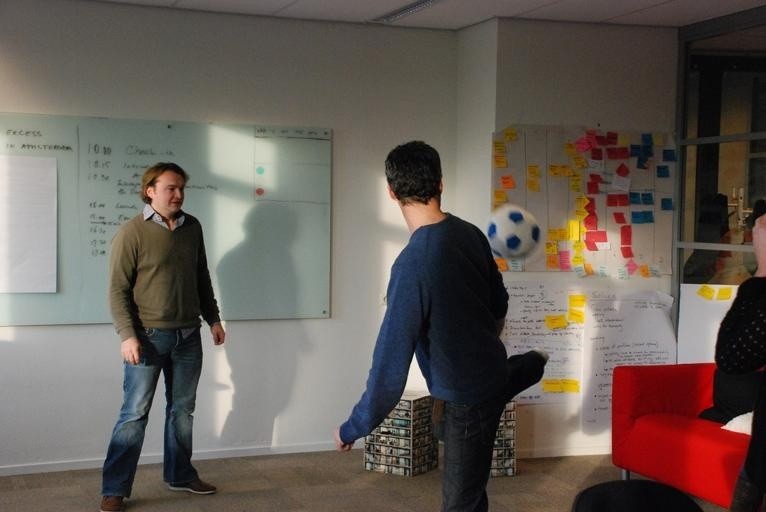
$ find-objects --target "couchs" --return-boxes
[611,361,752,508]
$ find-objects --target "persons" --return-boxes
[713,214,766,511]
[96,163,227,511]
[334,140,551,512]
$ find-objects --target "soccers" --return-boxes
[487,202,540,258]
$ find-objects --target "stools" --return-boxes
[572,480,703,512]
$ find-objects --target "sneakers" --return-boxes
[168,478,216,494]
[526,348,549,366]
[100,495,124,512]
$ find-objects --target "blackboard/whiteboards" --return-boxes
[0,110,333,326]
